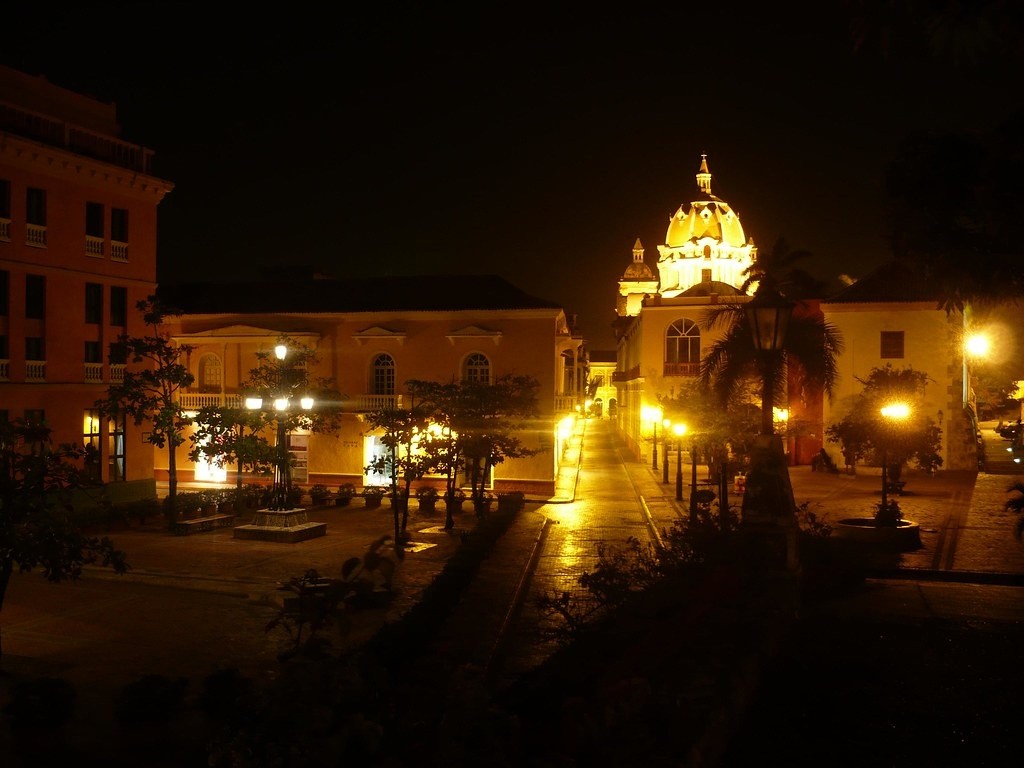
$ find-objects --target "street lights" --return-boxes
[674,424,686,503]
[661,418,671,484]
[649,407,663,471]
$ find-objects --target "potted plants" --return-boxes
[285,487,307,506]
[389,485,409,509]
[201,489,218,517]
[470,490,492,512]
[416,485,440,513]
[336,483,355,506]
[182,492,202,517]
[443,487,467,511]
[310,483,332,504]
[498,491,525,511]
[219,484,271,514]
[362,486,383,507]
